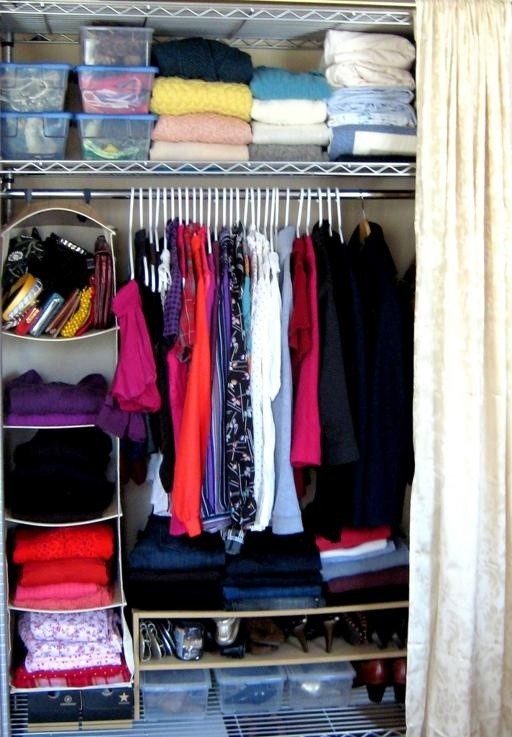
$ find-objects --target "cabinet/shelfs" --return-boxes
[135,600,410,735]
[0,206,137,736]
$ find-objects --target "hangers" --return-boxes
[114,187,394,300]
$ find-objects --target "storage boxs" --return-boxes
[0,24,160,161]
[140,659,356,721]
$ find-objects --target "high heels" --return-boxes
[138,608,409,704]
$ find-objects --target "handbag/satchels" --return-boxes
[1,227,114,338]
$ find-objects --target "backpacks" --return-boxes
[3,427,116,522]
